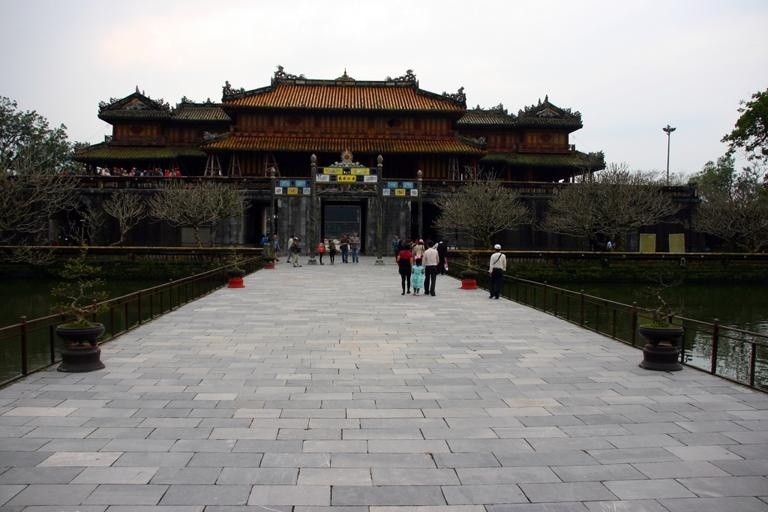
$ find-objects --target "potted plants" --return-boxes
[47,252,108,352]
[629,254,693,348]
[458,248,480,279]
[222,248,246,277]
[262,243,279,263]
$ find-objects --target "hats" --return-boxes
[494,244,502,250]
[419,239,424,243]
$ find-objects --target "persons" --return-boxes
[96,165,181,177]
[488,243,507,299]
[259,232,361,267]
[391,233,448,296]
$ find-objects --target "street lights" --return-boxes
[663,125,676,186]
